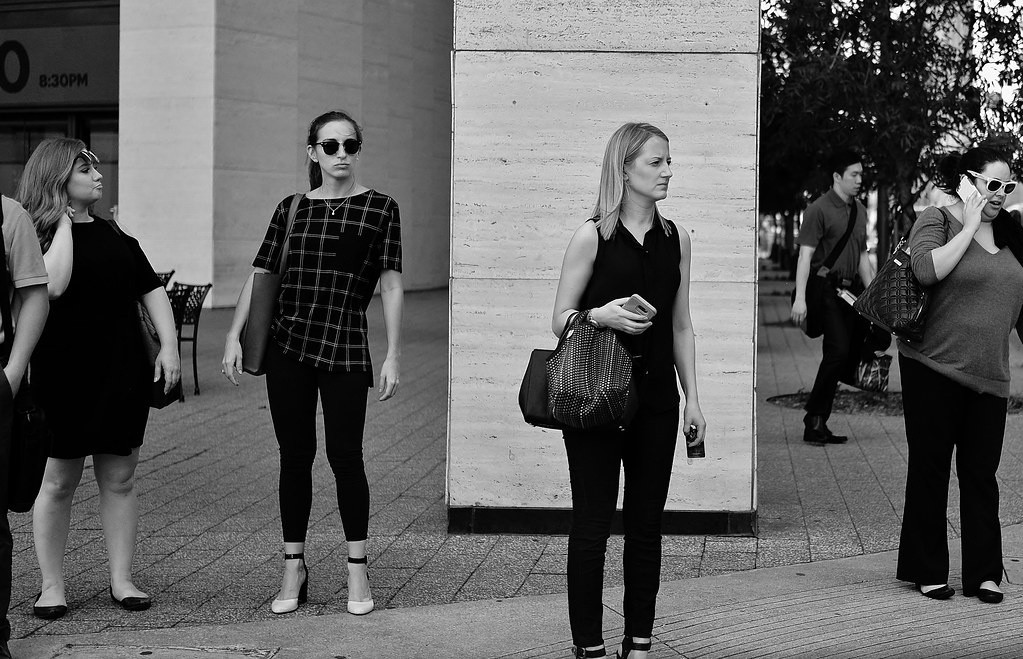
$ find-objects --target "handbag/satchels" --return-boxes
[130,331,182,409]
[840,353,892,394]
[853,208,949,341]
[546,309,633,428]
[518,312,579,427]
[242,193,306,376]
[0,376,46,512]
[791,264,835,338]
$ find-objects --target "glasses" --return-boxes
[312,139,362,156]
[967,169,1018,195]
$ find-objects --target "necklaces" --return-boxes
[322,185,357,215]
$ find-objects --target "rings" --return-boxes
[222,369,225,374]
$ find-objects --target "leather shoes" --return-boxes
[33,591,65,619]
[109,585,151,610]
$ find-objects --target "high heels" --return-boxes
[347,555,374,615]
[271,553,308,614]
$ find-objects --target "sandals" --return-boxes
[572,646,606,659]
[616,636,651,659]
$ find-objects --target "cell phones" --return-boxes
[622,294,656,323]
[956,177,984,205]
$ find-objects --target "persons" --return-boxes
[896,147,1023,605]
[222,111,405,615]
[551,123,707,659]
[12,137,182,617]
[0,195,52,659]
[791,151,874,444]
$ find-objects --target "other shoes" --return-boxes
[804,415,848,445]
[964,587,1003,604]
[915,584,955,600]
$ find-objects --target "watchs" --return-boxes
[587,308,607,330]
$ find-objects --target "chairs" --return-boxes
[166,281,213,401]
